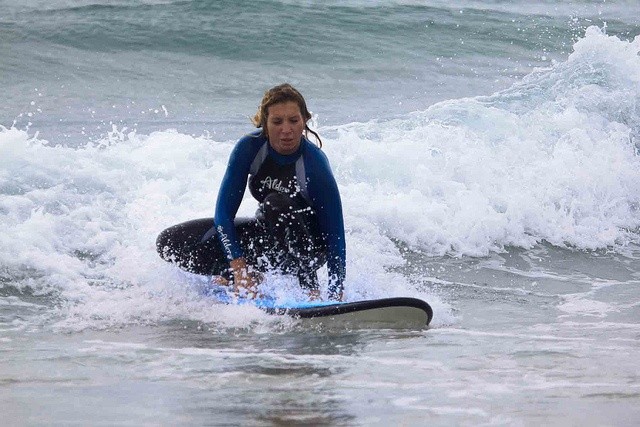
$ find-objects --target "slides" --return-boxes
[195,280,433,331]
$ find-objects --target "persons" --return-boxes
[155,83,347,304]
